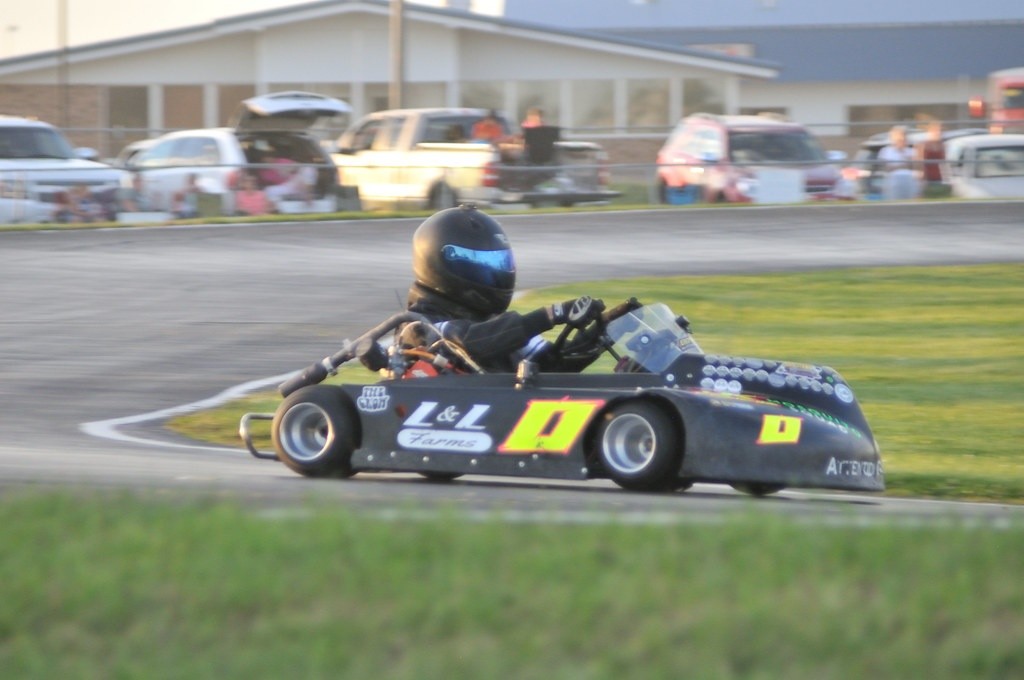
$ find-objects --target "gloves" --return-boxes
[609,300,644,332]
[551,296,606,329]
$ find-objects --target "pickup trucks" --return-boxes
[323,106,625,208]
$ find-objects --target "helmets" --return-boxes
[412,203,516,315]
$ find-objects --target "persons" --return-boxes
[389,202,605,378]
[52,169,266,224]
[521,107,561,165]
[260,156,320,212]
[473,109,507,144]
[876,120,947,183]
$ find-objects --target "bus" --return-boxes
[111,90,362,224]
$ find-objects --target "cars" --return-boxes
[938,133,1023,199]
[0,114,136,227]
[653,111,857,203]
